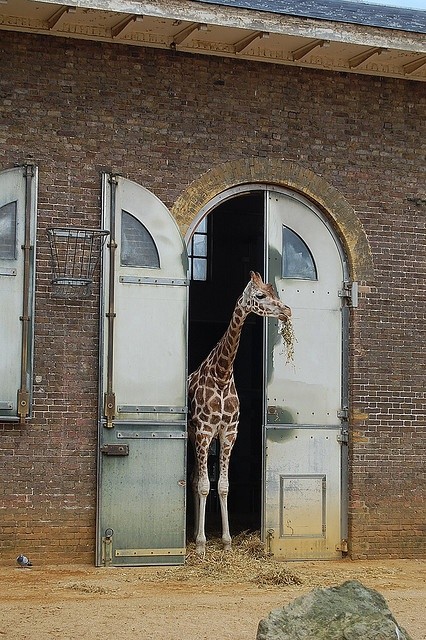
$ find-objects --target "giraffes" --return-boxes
[187,270,293,560]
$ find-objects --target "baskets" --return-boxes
[46,227,110,286]
